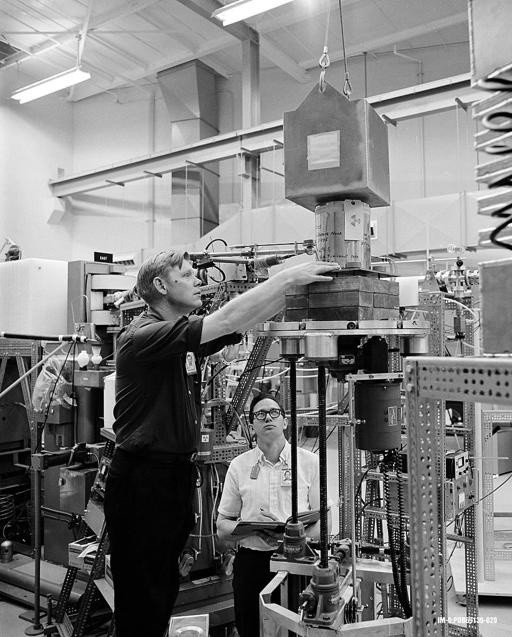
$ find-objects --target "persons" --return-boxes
[282,470,292,482]
[215,392,333,637]
[105,250,339,637]
[186,353,196,371]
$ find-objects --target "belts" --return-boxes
[116,450,199,466]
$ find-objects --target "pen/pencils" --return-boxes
[260,507,274,519]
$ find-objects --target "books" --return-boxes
[230,508,328,536]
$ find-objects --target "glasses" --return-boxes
[251,409,286,420]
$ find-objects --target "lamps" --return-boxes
[5,33,90,104]
[209,0,293,27]
